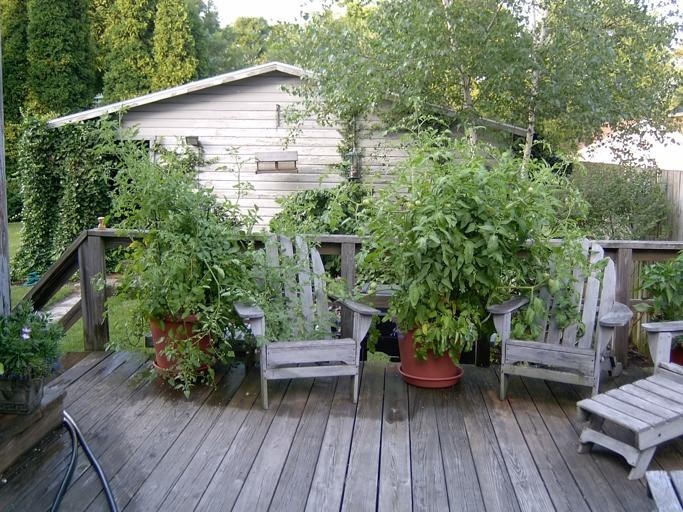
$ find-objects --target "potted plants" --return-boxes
[0,297,67,417]
[90,101,348,403]
[336,97,611,380]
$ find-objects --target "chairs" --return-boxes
[232,233,381,410]
